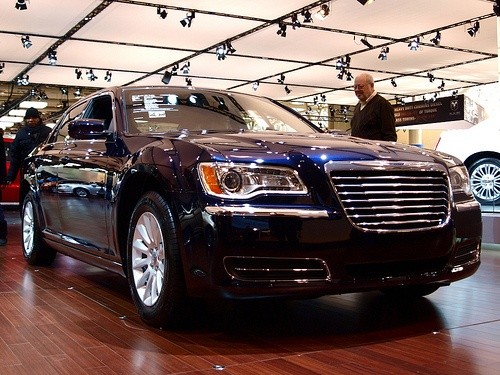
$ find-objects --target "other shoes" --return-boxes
[0,238,7,246]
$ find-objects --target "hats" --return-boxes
[24,107,40,120]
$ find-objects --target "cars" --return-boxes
[0,134,21,205]
[434,111,500,206]
[16,84,485,330]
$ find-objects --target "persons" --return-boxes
[349,72,397,141]
[0,126,7,245]
[7,106,53,222]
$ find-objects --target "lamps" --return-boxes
[466,20,480,37]
[0,0,234,100]
[252,0,458,116]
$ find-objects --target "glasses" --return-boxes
[353,82,371,89]
